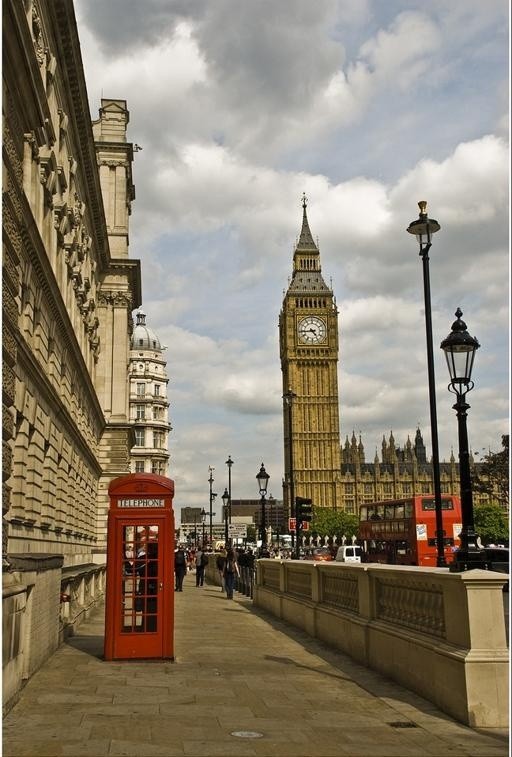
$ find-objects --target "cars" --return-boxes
[180,544,342,560]
[454,543,509,593]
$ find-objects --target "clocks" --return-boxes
[297,314,328,345]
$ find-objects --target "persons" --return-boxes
[125,541,157,633]
[124,541,301,600]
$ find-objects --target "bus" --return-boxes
[357,492,463,567]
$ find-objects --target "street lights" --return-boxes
[406,199,447,568]
[200,506,207,552]
[268,493,274,546]
[256,462,270,559]
[282,386,296,559]
[222,487,231,542]
[275,526,280,548]
[188,519,198,551]
[440,307,493,572]
[225,455,234,550]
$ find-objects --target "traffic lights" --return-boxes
[296,496,312,525]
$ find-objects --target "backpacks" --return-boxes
[200,553,209,566]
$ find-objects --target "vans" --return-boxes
[335,545,364,563]
